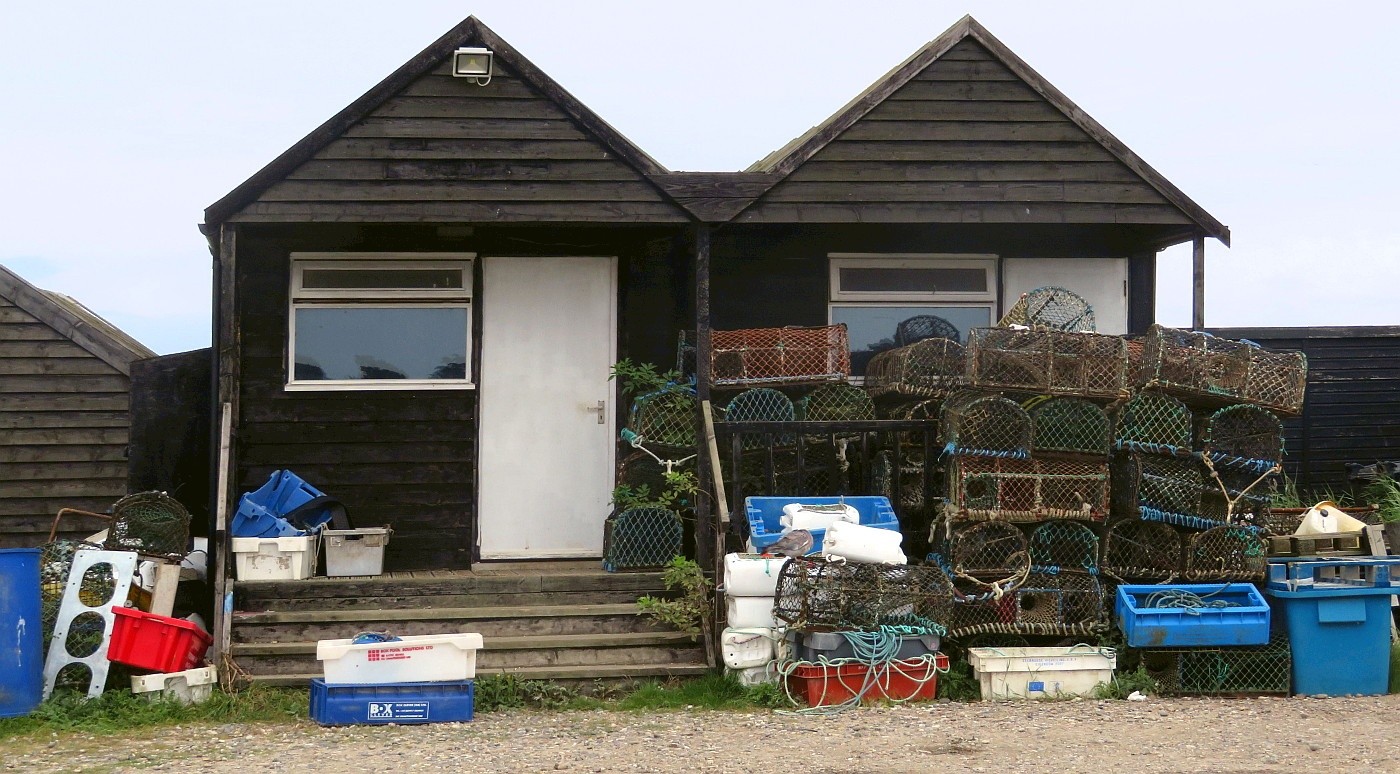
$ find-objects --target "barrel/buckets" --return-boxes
[822,520,907,566]
[0,548,44,718]
[780,504,860,533]
[721,536,919,688]
[1269,587,1400,695]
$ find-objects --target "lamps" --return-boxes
[452,47,493,84]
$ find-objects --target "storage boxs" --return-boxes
[790,632,940,665]
[744,496,900,553]
[43,577,152,611]
[1267,587,1400,697]
[231,469,333,537]
[310,678,474,726]
[1116,583,1270,645]
[774,655,949,709]
[316,632,484,683]
[232,536,318,581]
[969,648,1117,700]
[131,666,218,707]
[322,527,388,576]
[107,606,213,672]
